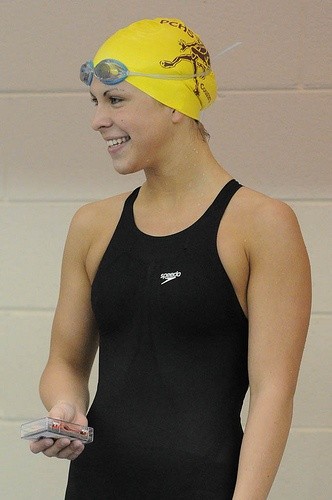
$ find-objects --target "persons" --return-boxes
[29,18,311,500]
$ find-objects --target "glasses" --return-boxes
[79,58,206,85]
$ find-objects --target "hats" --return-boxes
[93,18,217,121]
